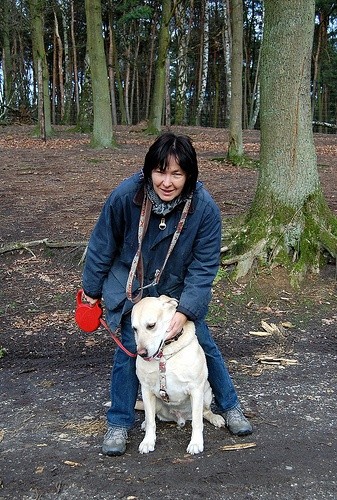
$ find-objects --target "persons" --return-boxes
[80,131,254,457]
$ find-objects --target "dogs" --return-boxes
[131,295,226,455]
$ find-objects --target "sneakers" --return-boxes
[223,402,252,436]
[102,427,127,455]
